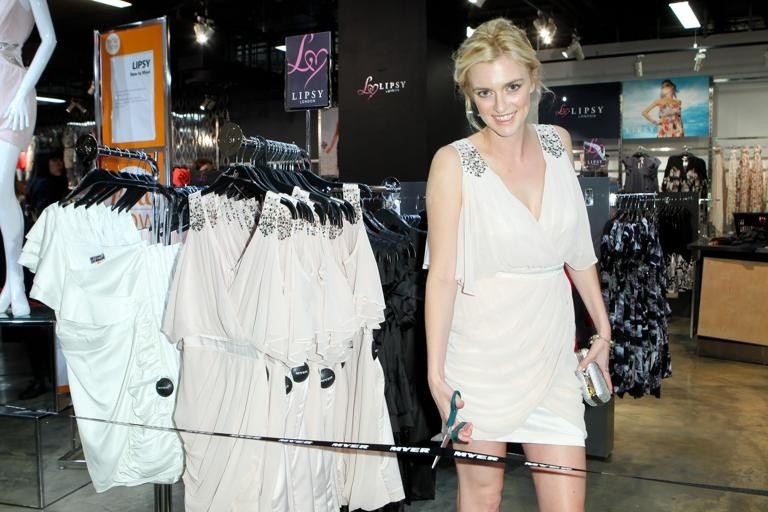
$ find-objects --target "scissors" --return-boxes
[431,390,467,469]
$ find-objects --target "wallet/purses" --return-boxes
[572,348,611,396]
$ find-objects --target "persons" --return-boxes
[1,1,60,328]
[640,78,685,139]
[28,153,66,220]
[189,158,214,173]
[413,17,616,512]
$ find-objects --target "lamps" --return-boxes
[192,0,217,46]
[200,87,218,112]
[66,79,95,114]
[533,10,708,78]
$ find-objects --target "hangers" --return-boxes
[613,192,697,231]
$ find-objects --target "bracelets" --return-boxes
[588,332,615,349]
